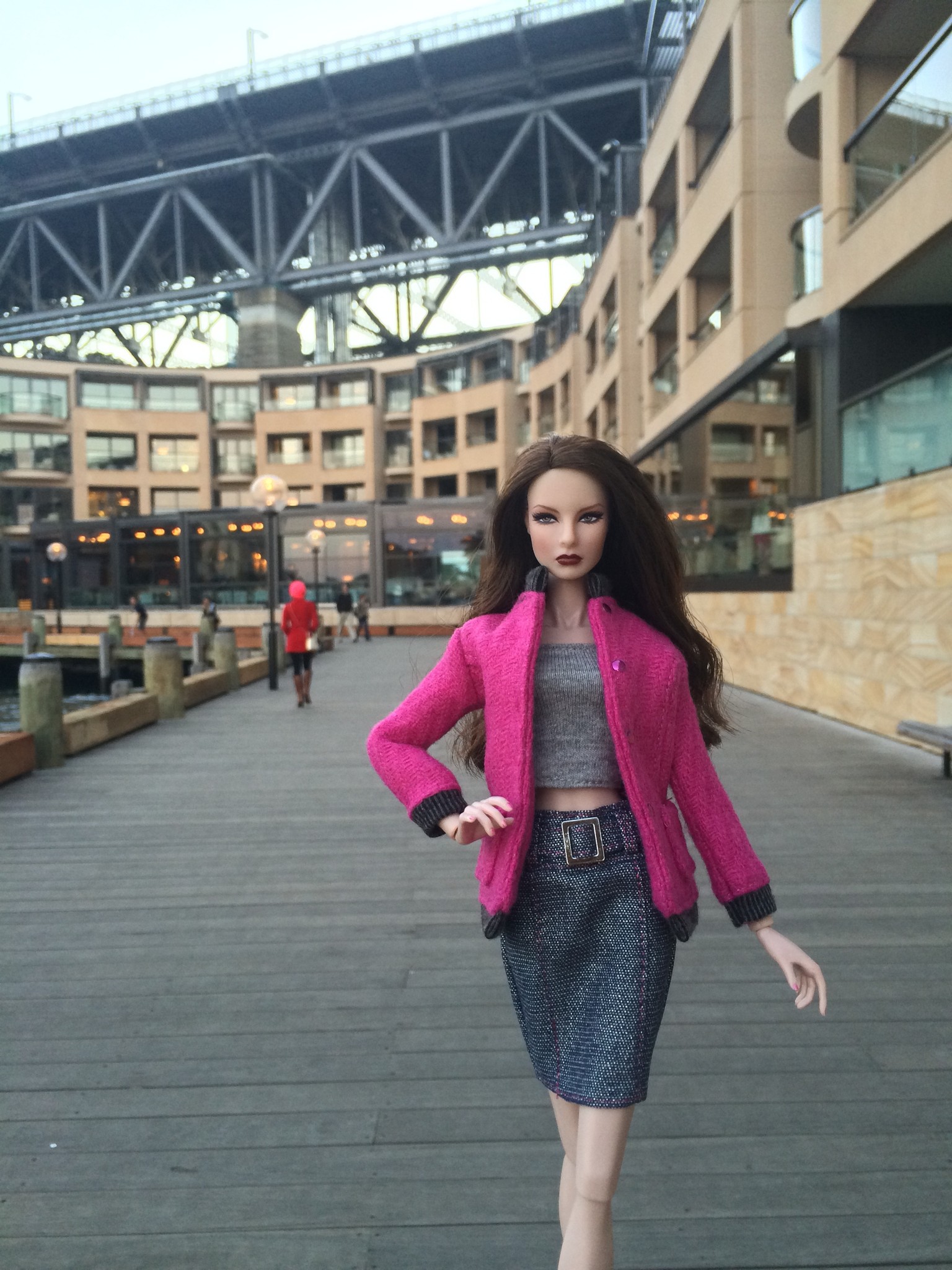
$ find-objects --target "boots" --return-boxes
[303,669,312,703]
[294,674,304,707]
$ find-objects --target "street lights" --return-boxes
[306,528,326,610]
[249,473,288,693]
[46,541,69,636]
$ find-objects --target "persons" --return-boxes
[367,437,828,1270]
[130,597,148,630]
[336,583,359,643]
[201,597,218,634]
[282,580,321,708]
[354,594,370,641]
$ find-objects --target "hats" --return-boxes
[289,581,306,599]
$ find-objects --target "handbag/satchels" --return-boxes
[305,636,320,652]
